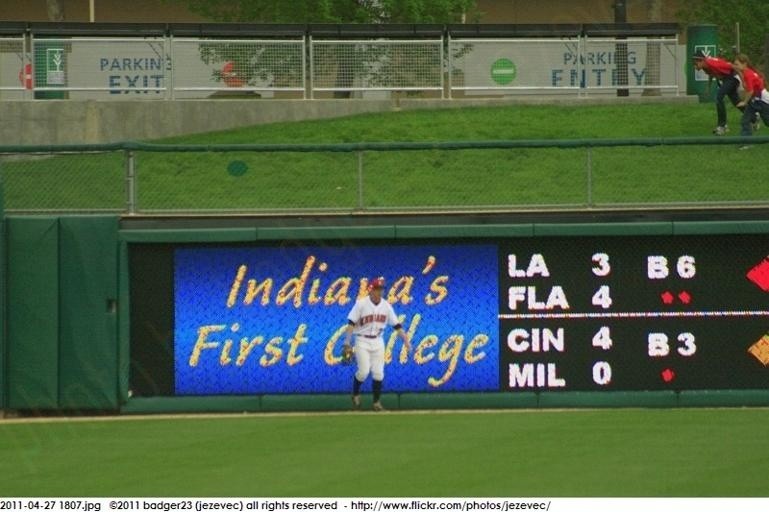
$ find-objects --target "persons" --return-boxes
[692,50,745,135]
[343,275,410,412]
[731,52,769,151]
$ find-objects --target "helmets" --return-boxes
[365,275,387,295]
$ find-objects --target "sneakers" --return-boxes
[751,111,762,131]
[351,393,362,408]
[371,400,387,413]
[713,124,732,136]
